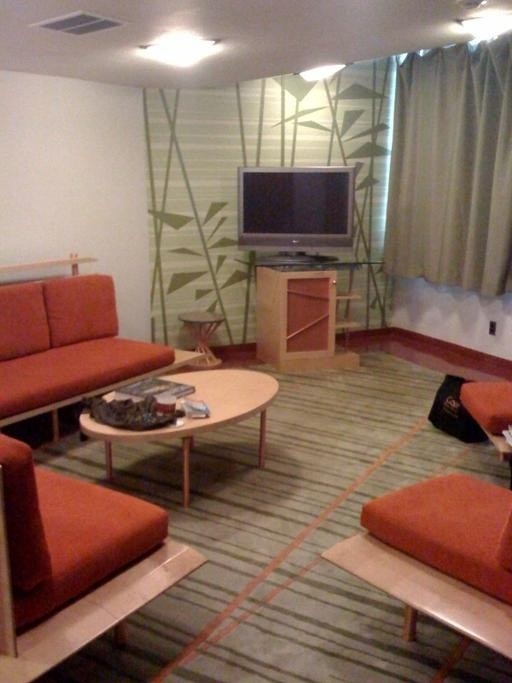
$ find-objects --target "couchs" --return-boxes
[0,276,180,428]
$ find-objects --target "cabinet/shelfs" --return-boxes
[256,265,361,374]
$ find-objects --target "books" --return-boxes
[115,376,195,403]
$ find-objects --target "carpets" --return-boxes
[0,352,512,680]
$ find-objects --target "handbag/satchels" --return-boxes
[428,374,490,442]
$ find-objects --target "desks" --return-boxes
[178,310,226,369]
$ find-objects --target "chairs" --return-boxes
[458,379,512,483]
[321,473,512,682]
[0,435,208,683]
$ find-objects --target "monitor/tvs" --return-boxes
[236,165,356,266]
